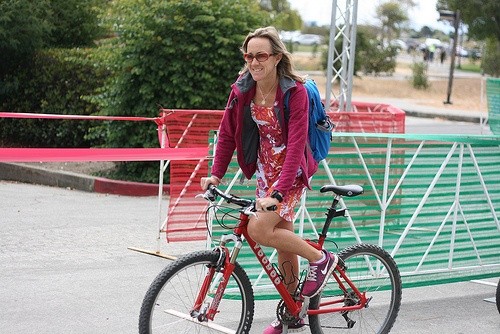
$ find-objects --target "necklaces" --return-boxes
[256,75,279,105]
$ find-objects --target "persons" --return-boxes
[420,44,447,64]
[200,25,339,334]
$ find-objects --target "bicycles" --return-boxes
[137,183,403,334]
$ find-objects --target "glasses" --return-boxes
[243,54,275,62]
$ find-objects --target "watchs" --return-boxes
[270,190,284,203]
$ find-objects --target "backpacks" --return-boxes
[283,79,334,161]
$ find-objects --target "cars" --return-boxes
[291,34,320,45]
[279,31,301,43]
[371,37,484,58]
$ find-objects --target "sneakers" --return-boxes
[262,312,305,334]
[302,250,338,298]
